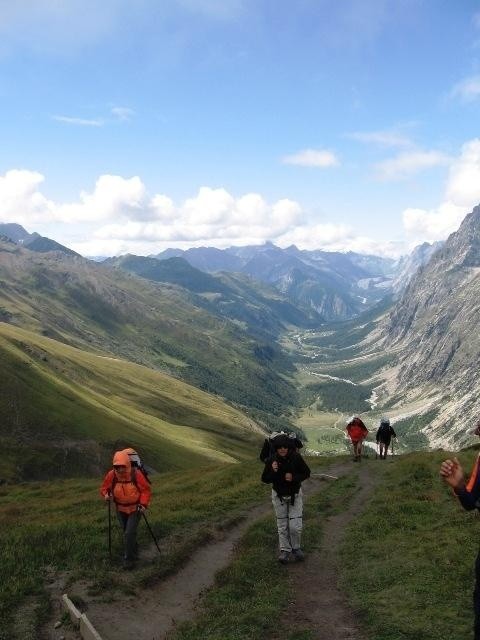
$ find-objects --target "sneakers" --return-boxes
[295,547,303,559]
[123,559,134,569]
[278,550,292,561]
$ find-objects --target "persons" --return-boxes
[441,420,480,639]
[346,417,368,461]
[100,451,152,571]
[261,435,311,562]
[376,418,396,459]
